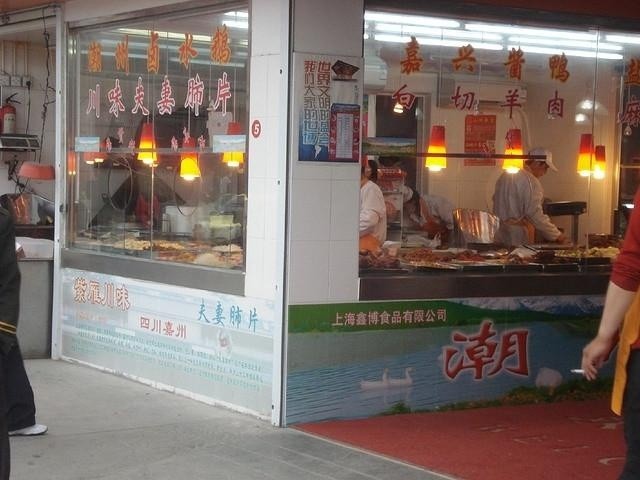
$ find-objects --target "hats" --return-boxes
[528,148,558,172]
[403,185,413,203]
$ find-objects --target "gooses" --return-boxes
[390,367,416,385]
[358,368,392,390]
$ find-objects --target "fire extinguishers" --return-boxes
[1,92,21,134]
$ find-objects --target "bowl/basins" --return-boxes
[17,161,55,181]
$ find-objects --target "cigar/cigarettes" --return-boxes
[569,369,584,374]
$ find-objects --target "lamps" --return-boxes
[6,162,55,207]
[135,32,247,179]
[422,28,608,179]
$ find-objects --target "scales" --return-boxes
[0,134,41,149]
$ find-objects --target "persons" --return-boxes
[4,241,48,436]
[403,186,458,248]
[359,155,387,252]
[493,148,566,251]
[581,188,640,480]
[0,206,22,480]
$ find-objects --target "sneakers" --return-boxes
[8,424,48,436]
